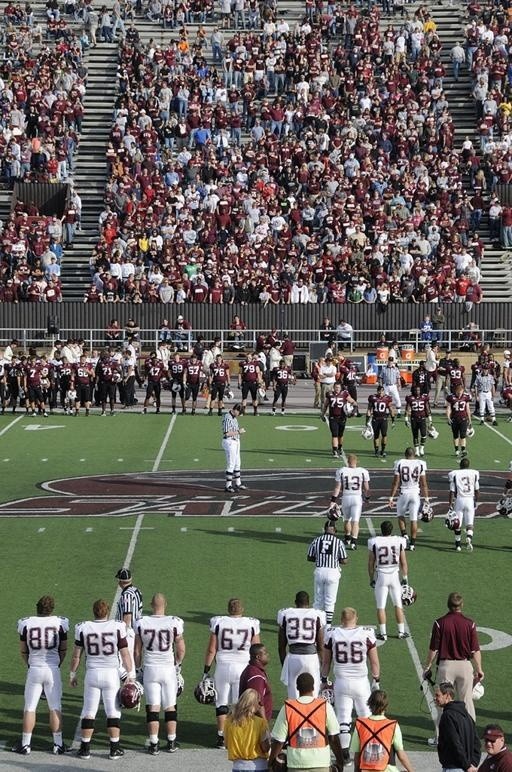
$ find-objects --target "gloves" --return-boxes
[202,673,209,680]
[70,672,77,688]
[175,661,181,672]
[372,677,380,692]
[136,667,143,680]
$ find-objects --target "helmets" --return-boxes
[466,427,475,437]
[172,382,181,392]
[361,425,374,440]
[418,502,434,522]
[176,669,185,697]
[327,503,341,521]
[194,677,217,704]
[496,498,512,514]
[427,426,439,440]
[318,681,335,705]
[114,372,122,383]
[343,402,354,417]
[401,585,417,606]
[68,388,77,399]
[257,388,265,399]
[41,377,50,389]
[199,371,209,382]
[445,509,460,530]
[224,389,234,400]
[117,681,144,709]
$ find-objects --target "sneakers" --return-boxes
[473,400,512,426]
[167,740,180,752]
[456,537,473,552]
[403,534,415,551]
[0,398,134,417]
[109,746,124,760]
[345,540,357,550]
[377,634,388,641]
[225,485,247,493]
[214,733,226,750]
[332,447,344,458]
[455,449,467,458]
[385,413,402,418]
[53,743,72,754]
[376,452,386,457]
[434,402,447,408]
[398,632,409,639]
[11,743,31,755]
[415,450,424,457]
[77,749,90,759]
[342,748,352,766]
[148,741,160,755]
[427,737,439,747]
[141,402,285,416]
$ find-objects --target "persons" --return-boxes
[365,386,396,457]
[421,592,483,747]
[445,384,472,458]
[448,459,480,553]
[115,570,143,683]
[268,673,345,772]
[388,447,430,551]
[204,598,262,749]
[328,454,370,549]
[277,591,326,700]
[0,0,512,417]
[69,599,136,759]
[433,682,483,771]
[133,593,186,755]
[307,520,348,629]
[468,723,512,771]
[321,382,358,458]
[368,519,409,639]
[223,688,270,771]
[321,607,380,767]
[404,386,433,458]
[503,460,512,497]
[18,595,70,755]
[221,403,247,493]
[348,690,412,771]
[239,644,272,721]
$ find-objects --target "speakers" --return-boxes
[48,315,60,334]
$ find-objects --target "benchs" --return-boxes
[0,0,512,302]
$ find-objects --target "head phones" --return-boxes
[419,672,436,690]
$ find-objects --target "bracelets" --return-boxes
[365,496,370,500]
[388,496,394,500]
[425,497,429,502]
[331,496,337,502]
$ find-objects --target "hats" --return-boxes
[325,520,335,527]
[114,568,131,579]
[481,729,503,742]
[473,677,484,700]
